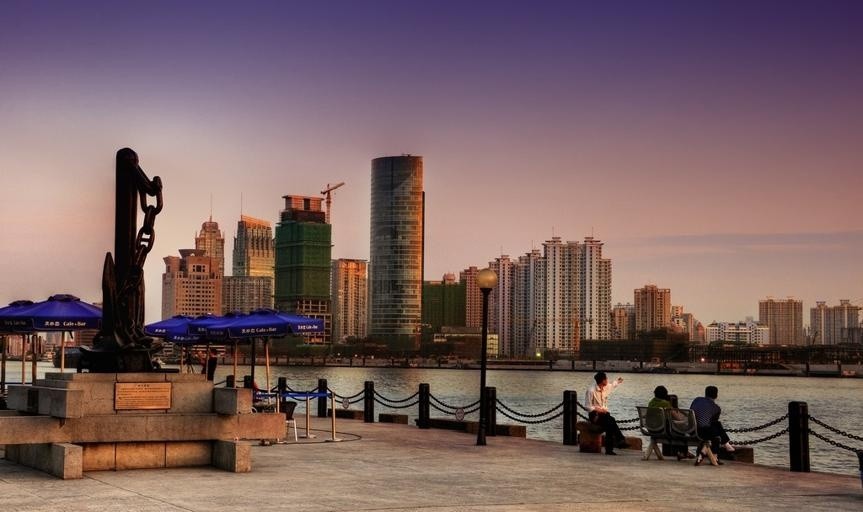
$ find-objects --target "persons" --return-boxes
[584,370,632,455]
[646,386,694,462]
[689,385,740,466]
[197,349,219,382]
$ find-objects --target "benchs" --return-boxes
[633,403,722,466]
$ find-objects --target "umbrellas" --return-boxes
[205,306,325,404]
[143,314,205,374]
[0,300,39,384]
[1,294,103,374]
[185,308,249,388]
[166,312,252,381]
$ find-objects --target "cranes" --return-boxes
[321,182,345,224]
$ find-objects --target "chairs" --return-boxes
[276,398,298,444]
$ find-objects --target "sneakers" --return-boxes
[710,458,723,465]
[677,451,694,460]
[728,449,744,456]
[605,439,630,455]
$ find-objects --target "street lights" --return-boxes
[476,268,498,445]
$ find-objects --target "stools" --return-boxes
[577,421,605,453]
